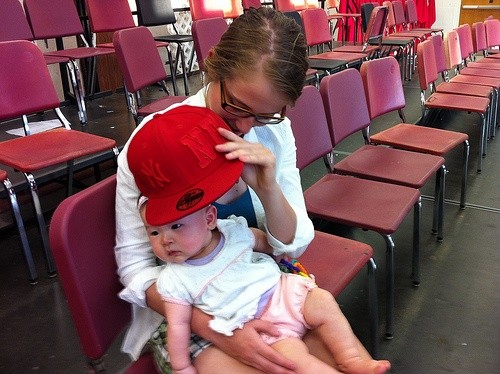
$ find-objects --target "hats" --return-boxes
[126,105,244,227]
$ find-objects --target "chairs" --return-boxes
[0,1,444,284]
[51,56,470,374]
[418,18,500,173]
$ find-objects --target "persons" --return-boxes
[138,190,391,374]
[113,6,373,374]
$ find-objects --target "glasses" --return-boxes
[219,77,288,125]
